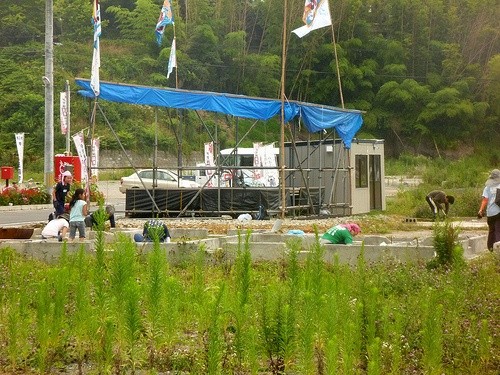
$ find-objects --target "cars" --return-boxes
[118,168,200,193]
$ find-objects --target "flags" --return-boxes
[90,137,102,185]
[249,140,280,188]
[203,141,217,188]
[72,130,89,187]
[89,0,104,98]
[290,0,335,39]
[166,36,178,79]
[14,132,28,186]
[59,91,69,136]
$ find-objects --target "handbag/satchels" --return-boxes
[495,188,500,207]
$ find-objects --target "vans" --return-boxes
[211,147,281,188]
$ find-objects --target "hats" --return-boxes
[484,168,500,185]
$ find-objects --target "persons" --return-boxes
[84,204,118,230]
[476,168,500,253]
[424,189,456,219]
[133,218,172,244]
[67,187,89,243]
[50,171,74,214]
[153,0,178,47]
[318,223,361,246]
[41,212,70,242]
